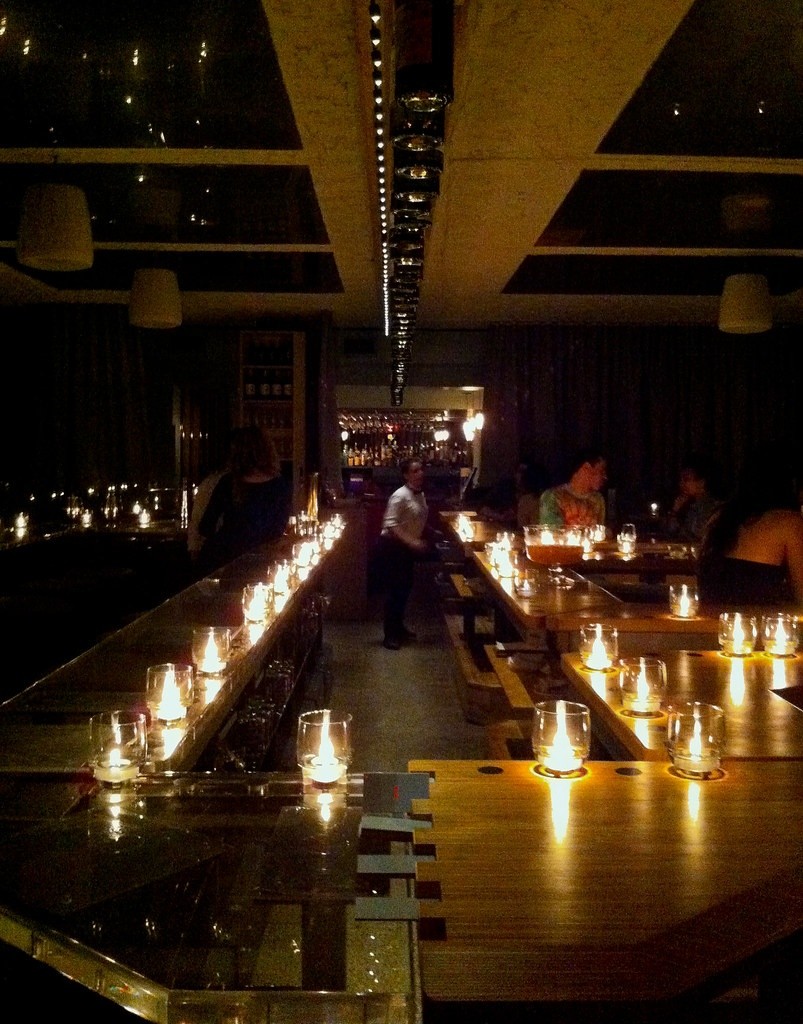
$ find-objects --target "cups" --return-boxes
[622,523,636,536]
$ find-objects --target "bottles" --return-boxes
[342,413,467,467]
[245,335,294,399]
[245,408,293,459]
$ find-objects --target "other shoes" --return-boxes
[383,635,400,650]
[398,628,417,638]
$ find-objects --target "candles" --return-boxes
[87,512,802,789]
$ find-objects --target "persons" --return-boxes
[666,461,727,546]
[188,426,287,584]
[380,460,443,650]
[699,438,803,612]
[539,449,606,525]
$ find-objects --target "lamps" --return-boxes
[717,273,773,335]
[132,269,184,328]
[14,157,90,270]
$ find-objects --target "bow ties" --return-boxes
[404,483,423,495]
